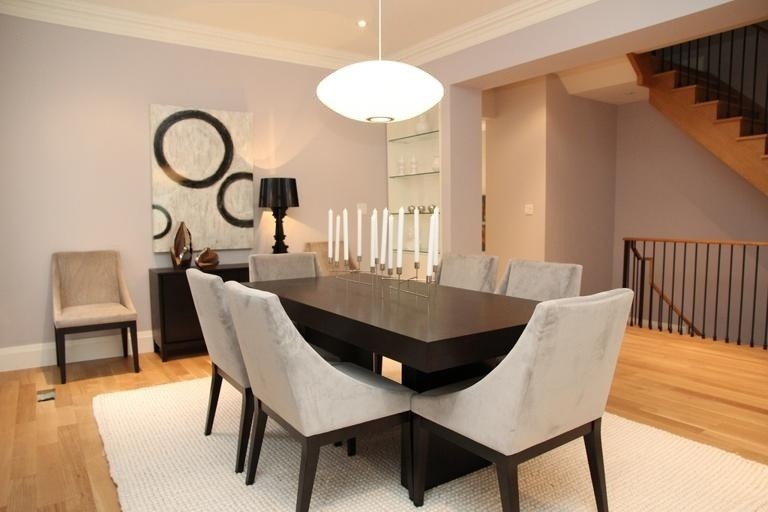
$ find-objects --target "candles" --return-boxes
[326,204,441,282]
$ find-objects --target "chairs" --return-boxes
[411,280,638,511]
[435,249,501,294]
[223,275,417,512]
[247,249,321,284]
[180,262,346,476]
[49,248,141,384]
[494,258,585,304]
[303,239,358,277]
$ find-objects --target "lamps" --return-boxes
[257,175,300,253]
[314,1,444,128]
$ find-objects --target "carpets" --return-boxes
[90,355,768,511]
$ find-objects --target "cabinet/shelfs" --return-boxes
[384,83,444,281]
[148,262,251,364]
